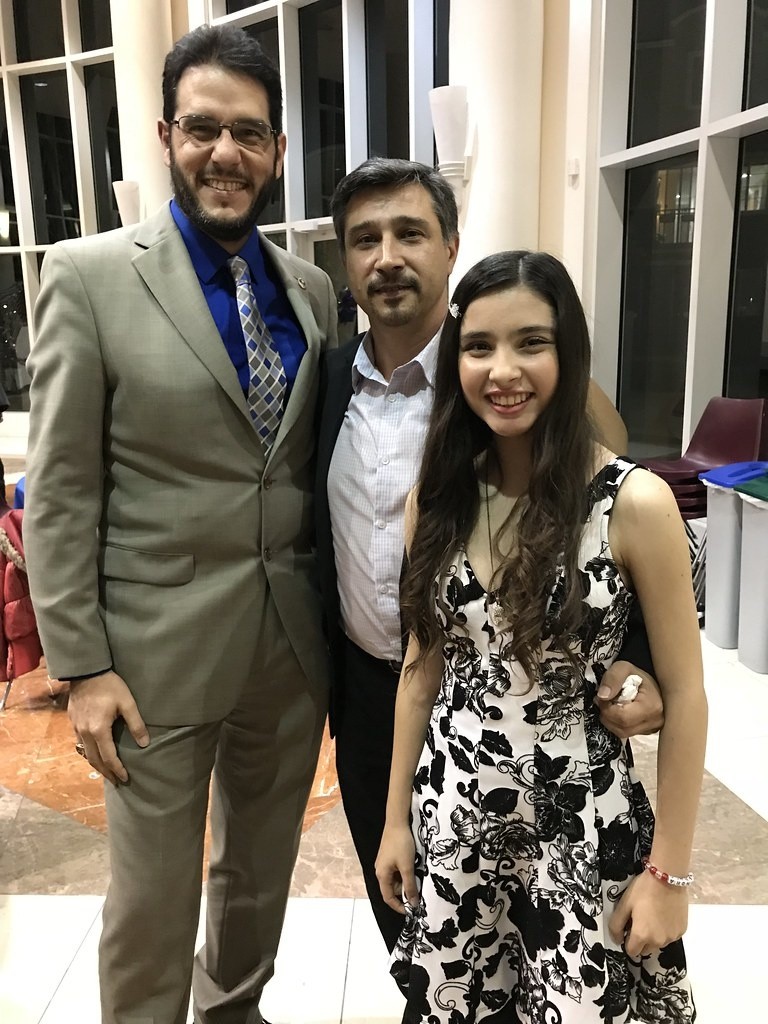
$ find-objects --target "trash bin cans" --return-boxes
[699,460,768,678]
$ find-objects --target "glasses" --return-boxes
[165,114,276,145]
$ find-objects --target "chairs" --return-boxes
[642,395,768,629]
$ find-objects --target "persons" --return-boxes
[23,27,343,1024]
[375,250,707,1024]
[310,155,665,952]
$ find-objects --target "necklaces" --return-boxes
[486,451,504,624]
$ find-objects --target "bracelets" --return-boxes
[642,855,695,887]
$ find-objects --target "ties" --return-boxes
[226,252,289,466]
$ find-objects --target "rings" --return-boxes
[75,743,87,758]
